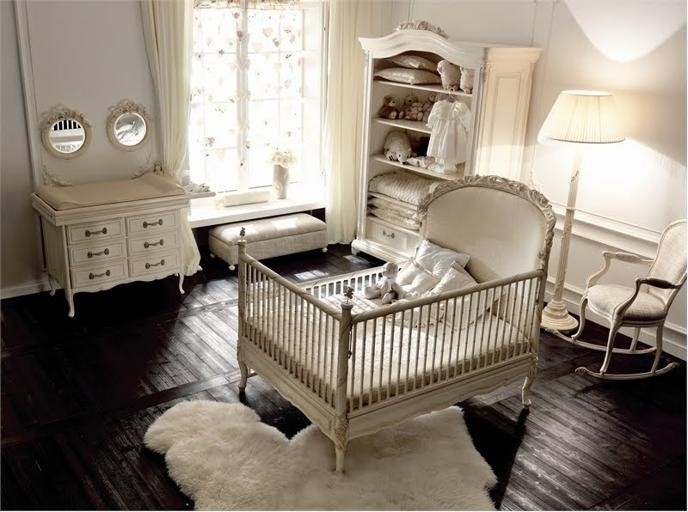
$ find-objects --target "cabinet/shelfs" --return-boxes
[30,171,192,318]
[350,17,543,268]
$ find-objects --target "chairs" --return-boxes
[545,217,688,381]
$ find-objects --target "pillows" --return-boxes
[397,238,508,334]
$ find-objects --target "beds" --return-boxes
[235,174,557,475]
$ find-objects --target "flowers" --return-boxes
[270,144,300,169]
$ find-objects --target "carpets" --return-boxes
[142,397,500,511]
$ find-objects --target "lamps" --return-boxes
[536,89,628,332]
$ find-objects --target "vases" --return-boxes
[271,164,289,200]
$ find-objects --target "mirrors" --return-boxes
[40,99,155,161]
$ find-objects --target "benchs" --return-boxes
[207,213,330,271]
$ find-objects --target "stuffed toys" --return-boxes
[363,262,405,304]
[377,60,474,123]
[381,132,417,165]
